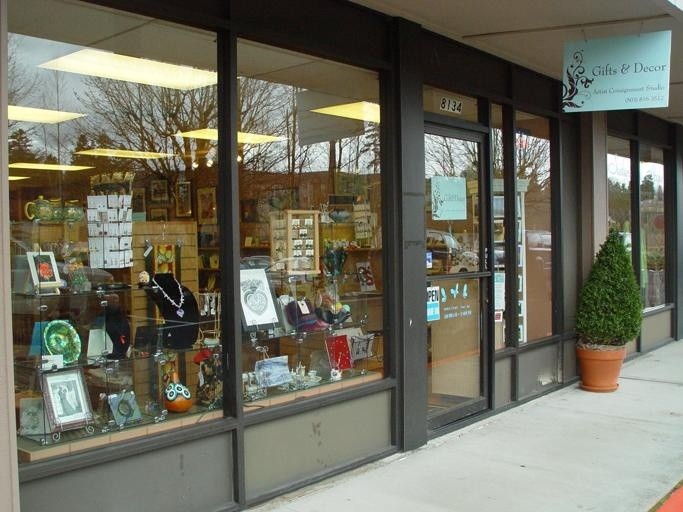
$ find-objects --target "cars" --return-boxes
[426,229,551,275]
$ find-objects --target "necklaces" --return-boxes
[152,276,185,318]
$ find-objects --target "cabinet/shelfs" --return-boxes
[196,244,268,294]
[266,208,322,280]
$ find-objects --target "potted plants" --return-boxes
[570,220,646,396]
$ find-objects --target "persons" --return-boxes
[58,384,79,415]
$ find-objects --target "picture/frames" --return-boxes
[24,247,60,286]
[34,363,96,435]
[131,175,217,227]
[238,264,284,334]
